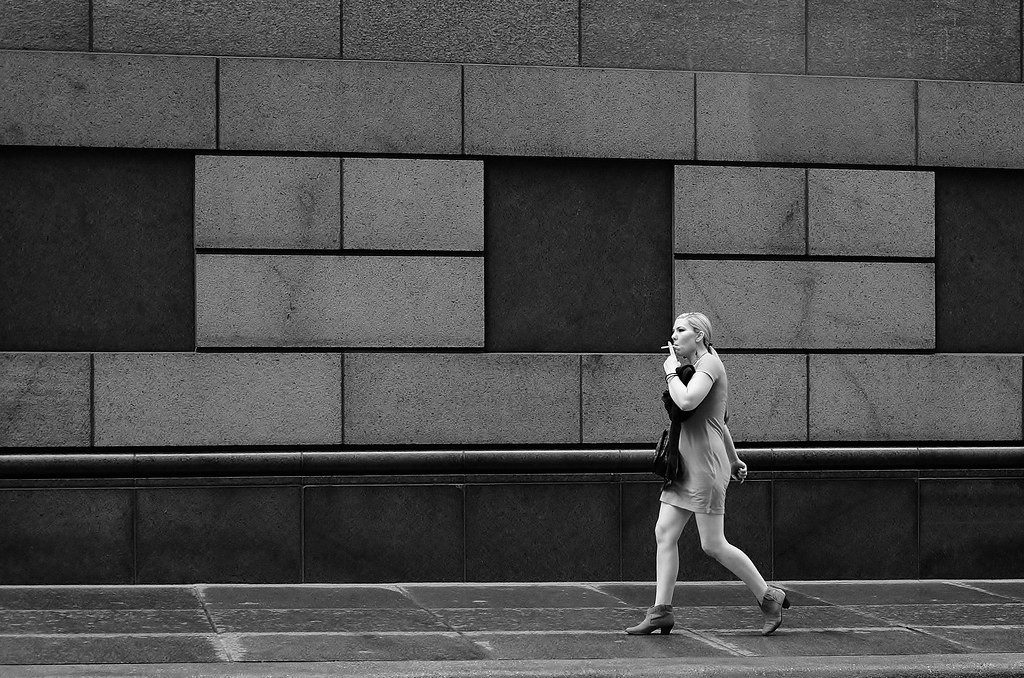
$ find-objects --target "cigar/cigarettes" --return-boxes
[661,344,675,349]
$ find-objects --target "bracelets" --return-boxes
[665,373,678,384]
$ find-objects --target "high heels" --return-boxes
[625,605,675,635]
[758,587,791,636]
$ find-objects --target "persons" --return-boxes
[625,312,791,635]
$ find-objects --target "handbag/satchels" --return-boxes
[651,428,685,482]
